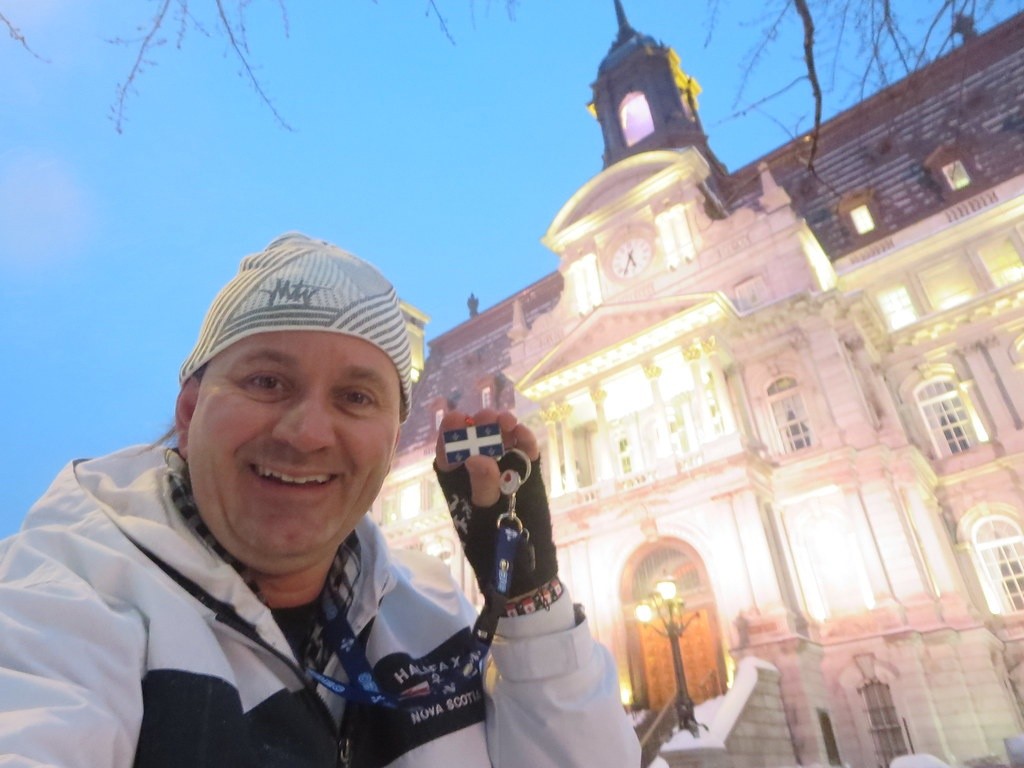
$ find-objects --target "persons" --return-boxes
[0,229,644,768]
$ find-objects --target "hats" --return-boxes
[178,231,415,427]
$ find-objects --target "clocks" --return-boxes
[611,235,653,278]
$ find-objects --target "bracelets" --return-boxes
[498,577,563,621]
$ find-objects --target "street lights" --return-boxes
[632,578,711,738]
